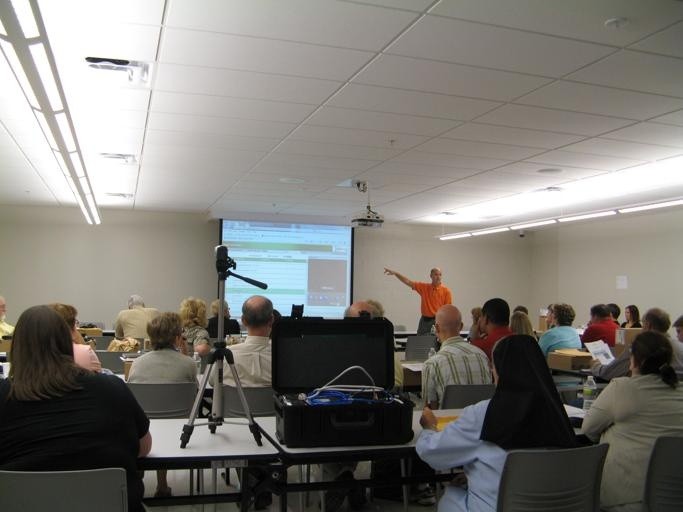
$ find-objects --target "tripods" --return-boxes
[180,245,267,449]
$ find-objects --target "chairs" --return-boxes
[0,333,13,362]
[404,336,438,360]
[78,328,151,382]
[440,384,497,410]
[0,468,127,512]
[127,381,198,497]
[217,382,277,485]
[496,442,610,511]
[644,431,683,512]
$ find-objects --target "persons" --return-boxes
[383,267,454,336]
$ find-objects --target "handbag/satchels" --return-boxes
[107,336,141,353]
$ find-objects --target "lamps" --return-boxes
[0,0,104,226]
[433,187,683,240]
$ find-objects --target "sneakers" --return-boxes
[254,485,273,510]
[399,485,436,506]
[318,470,370,512]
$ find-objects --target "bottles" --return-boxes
[581,375,598,417]
[427,347,435,358]
[191,350,201,380]
[224,333,246,346]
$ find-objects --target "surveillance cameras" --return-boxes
[519,234,525,238]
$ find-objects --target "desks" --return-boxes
[252,408,461,511]
[141,416,288,509]
[549,365,614,409]
[459,331,471,339]
[394,360,425,399]
[394,330,418,352]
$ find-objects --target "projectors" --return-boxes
[352,219,384,228]
